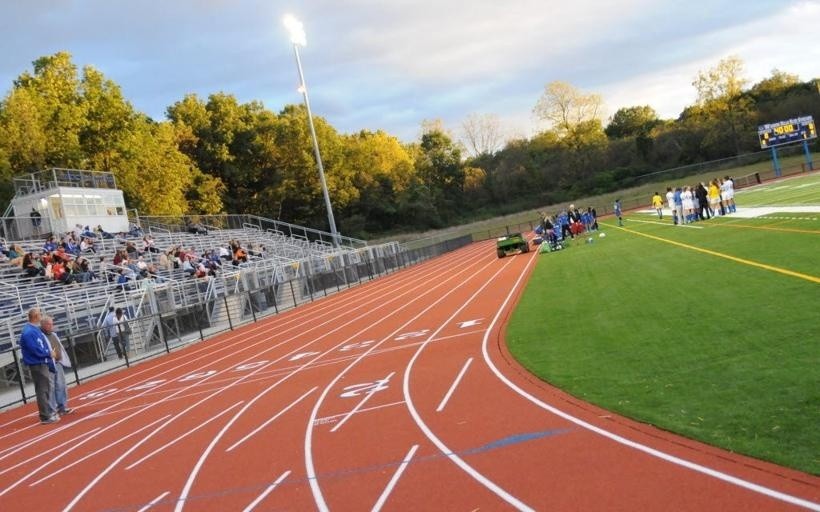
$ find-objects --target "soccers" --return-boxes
[599,233,605,238]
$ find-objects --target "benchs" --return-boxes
[0,233,242,356]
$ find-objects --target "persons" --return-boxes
[112,308,133,360]
[614,198,624,227]
[539,202,599,251]
[20,306,61,422]
[39,317,79,416]
[664,176,737,227]
[104,306,122,359]
[30,206,43,235]
[652,192,662,218]
[0,224,268,286]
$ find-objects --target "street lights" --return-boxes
[278,12,341,252]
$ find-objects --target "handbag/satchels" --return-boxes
[123,315,132,334]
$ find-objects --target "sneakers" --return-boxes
[56,407,70,412]
[39,414,61,423]
